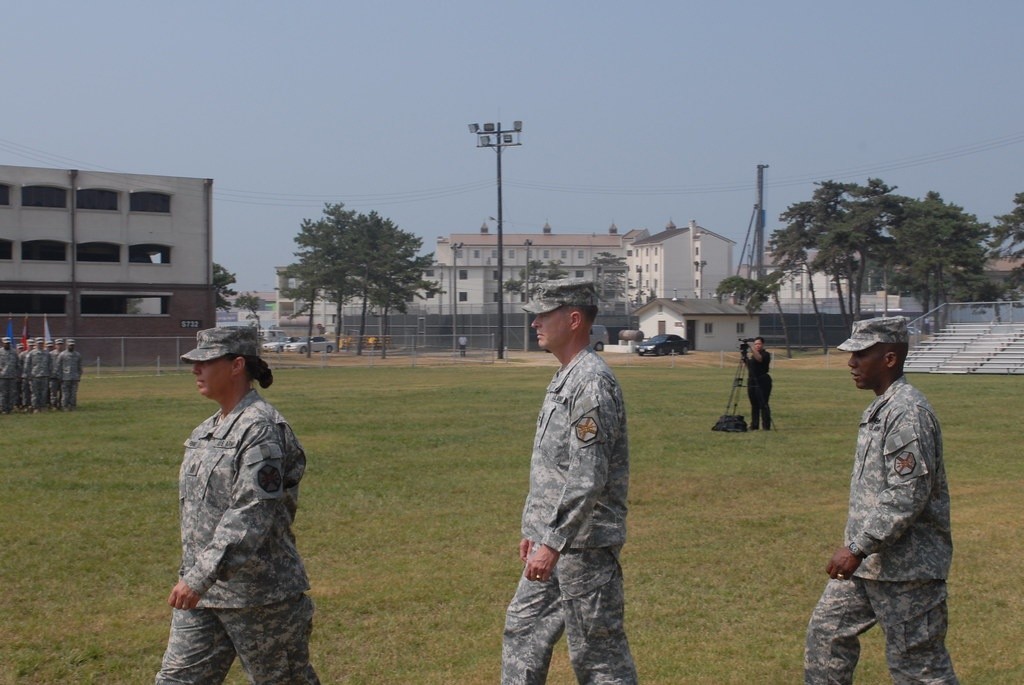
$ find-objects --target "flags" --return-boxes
[21,317,30,351]
[7,317,16,349]
[44,316,52,343]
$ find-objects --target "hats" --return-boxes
[1,337,11,344]
[26,340,36,346]
[836,316,909,352]
[521,277,598,314]
[46,341,54,346]
[180,326,258,363]
[55,339,64,344]
[66,339,76,345]
[35,337,45,344]
[15,344,24,349]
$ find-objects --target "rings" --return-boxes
[536,575,541,581]
[837,574,844,581]
[182,605,189,609]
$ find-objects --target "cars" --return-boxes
[589,324,609,351]
[284,335,337,354]
[635,334,690,357]
[259,335,306,353]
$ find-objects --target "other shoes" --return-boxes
[749,426,759,430]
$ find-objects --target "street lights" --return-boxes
[466,119,524,361]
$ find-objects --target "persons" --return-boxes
[744,337,773,431]
[0,337,83,413]
[802,316,961,685]
[459,334,468,356]
[499,277,640,685]
[156,326,322,685]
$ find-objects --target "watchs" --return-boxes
[848,542,867,559]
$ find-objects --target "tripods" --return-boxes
[725,350,777,431]
